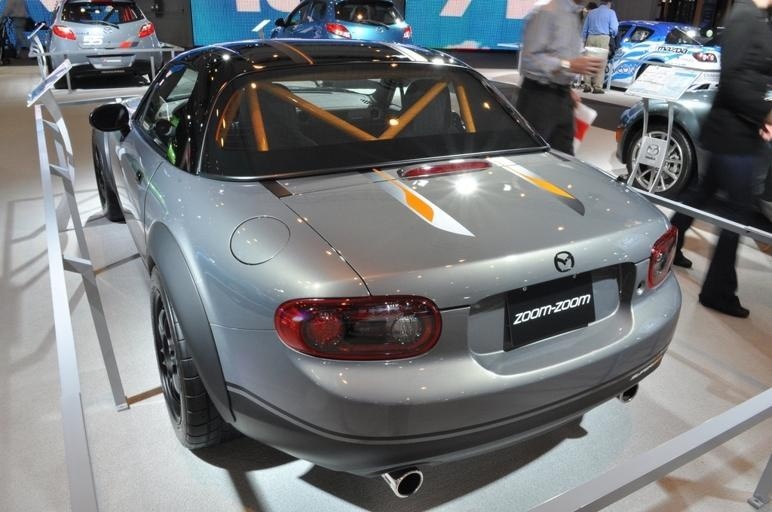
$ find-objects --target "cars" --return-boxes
[88,37,683,500]
[615,86,771,223]
[606,20,721,91]
[269,0,412,46]
[35,2,163,89]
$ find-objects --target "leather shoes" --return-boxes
[583,85,604,94]
[699,295,749,318]
[673,251,692,268]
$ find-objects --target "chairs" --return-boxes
[226,82,319,152]
[376,79,462,141]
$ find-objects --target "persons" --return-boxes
[667,0,772,317]
[517,0,618,155]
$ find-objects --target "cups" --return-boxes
[586,46,609,58]
